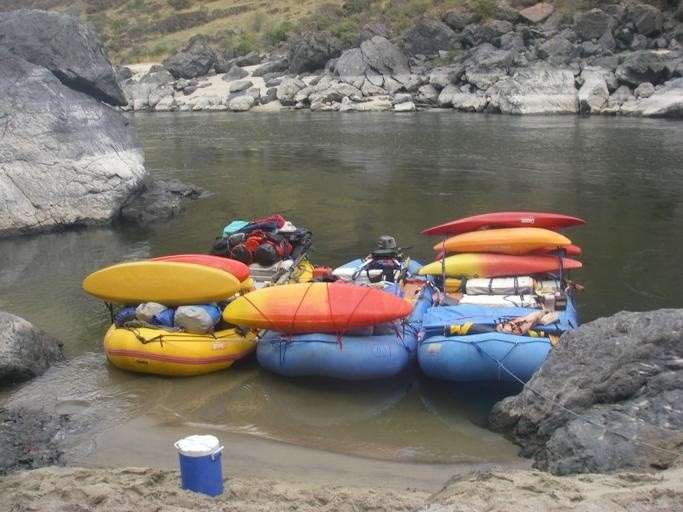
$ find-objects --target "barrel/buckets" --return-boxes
[175,435,223,496]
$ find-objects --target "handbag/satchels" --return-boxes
[114,301,234,334]
[209,213,292,266]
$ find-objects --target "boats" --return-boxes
[80,260,242,308]
[257,233,436,386]
[416,272,581,383]
[422,211,586,237]
[432,226,572,256]
[145,254,251,283]
[523,245,583,257]
[418,252,584,280]
[103,254,314,378]
[222,280,413,334]
